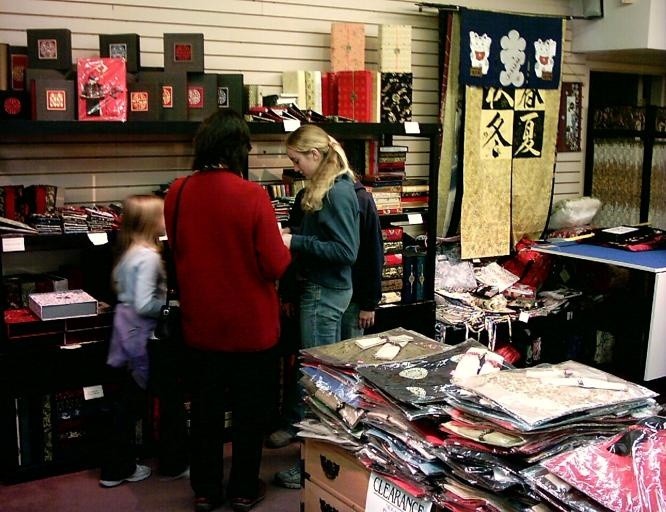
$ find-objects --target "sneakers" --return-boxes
[98,428,301,510]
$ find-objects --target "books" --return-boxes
[0,200,122,237]
[380,228,425,303]
[243,69,333,126]
[263,171,308,224]
[366,145,431,216]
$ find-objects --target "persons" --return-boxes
[266,167,386,448]
[271,123,361,493]
[164,107,288,509]
[96,197,175,488]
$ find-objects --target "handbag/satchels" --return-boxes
[154,305,184,342]
[435,234,582,327]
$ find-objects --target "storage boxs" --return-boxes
[279,20,421,125]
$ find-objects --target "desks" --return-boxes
[530,233,665,388]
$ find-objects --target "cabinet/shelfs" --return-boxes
[291,425,375,512]
[0,118,435,490]
